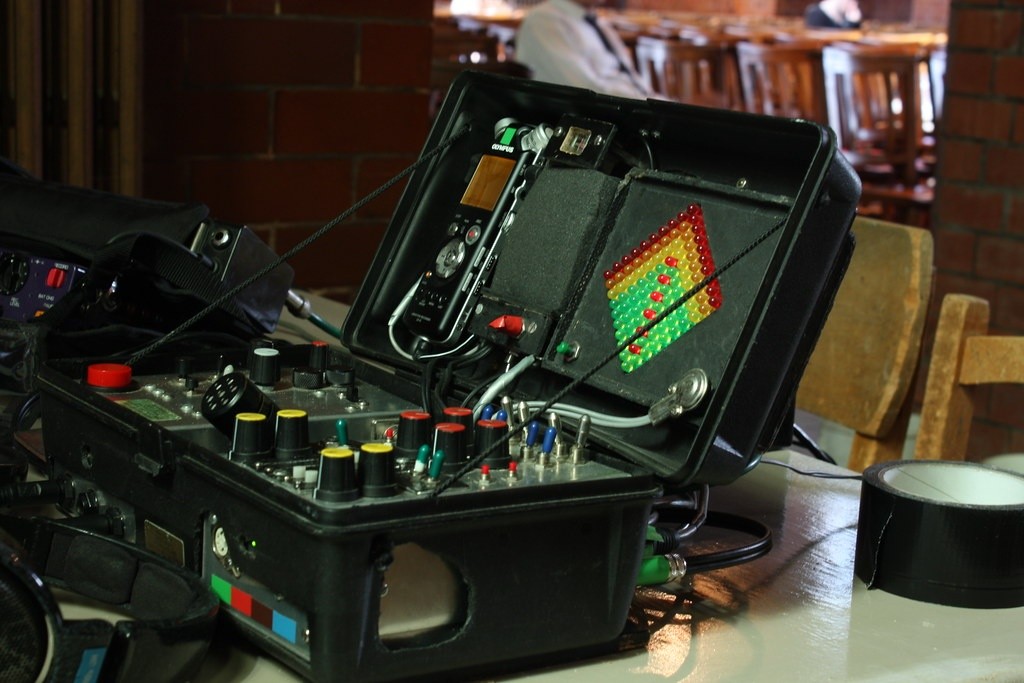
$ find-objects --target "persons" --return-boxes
[805,0,864,48]
[514,0,672,102]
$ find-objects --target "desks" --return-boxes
[433,0,946,131]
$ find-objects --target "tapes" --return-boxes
[855,458,1024,610]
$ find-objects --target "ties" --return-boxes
[582,11,646,96]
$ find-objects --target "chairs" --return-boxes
[795,213,935,461]
[913,297,1024,462]
[629,26,938,212]
